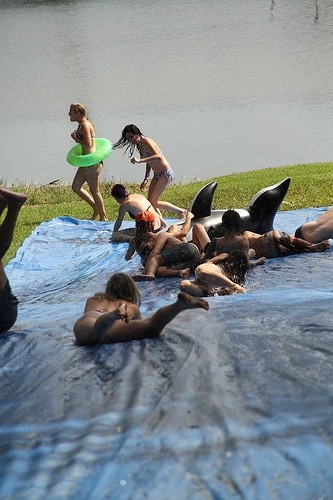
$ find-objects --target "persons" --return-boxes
[113,124,190,228]
[0,188,29,332]
[66,103,112,223]
[109,184,332,298]
[72,273,210,347]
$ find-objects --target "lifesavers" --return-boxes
[65,137,112,167]
